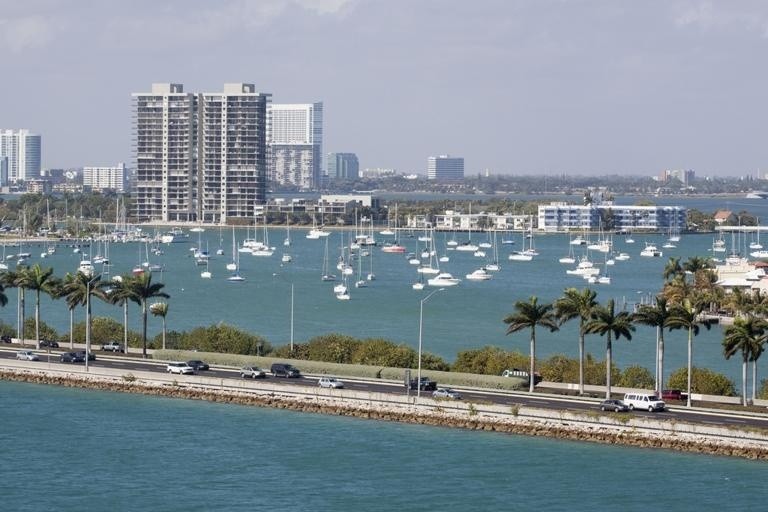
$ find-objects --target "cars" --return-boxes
[167,358,210,374]
[406,375,465,402]
[15,351,39,361]
[317,379,344,390]
[599,399,629,413]
[59,350,98,363]
[39,339,60,348]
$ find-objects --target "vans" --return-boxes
[655,389,682,402]
[623,393,666,412]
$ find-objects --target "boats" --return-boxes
[160,227,190,243]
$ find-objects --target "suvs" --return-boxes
[240,365,265,379]
[271,364,300,378]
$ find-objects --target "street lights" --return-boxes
[84,270,107,374]
[416,288,446,401]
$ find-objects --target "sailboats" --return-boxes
[408,211,540,291]
[217,222,245,282]
[0,203,32,271]
[748,217,768,257]
[37,199,58,258]
[306,211,332,240]
[190,204,213,279]
[135,242,164,274]
[322,238,336,282]
[281,214,294,264]
[638,209,680,258]
[333,203,405,299]
[559,212,633,286]
[63,205,110,279]
[115,198,164,243]
[238,202,278,257]
[707,220,727,253]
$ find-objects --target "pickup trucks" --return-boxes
[102,342,125,352]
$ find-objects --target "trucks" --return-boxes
[501,367,544,386]
